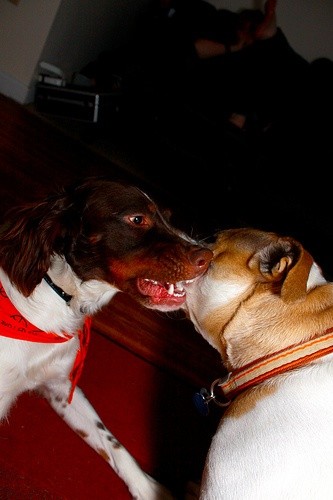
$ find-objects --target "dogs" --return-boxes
[183,225,333,500]
[1,174,215,422]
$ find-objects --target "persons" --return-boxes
[83,0,333,280]
